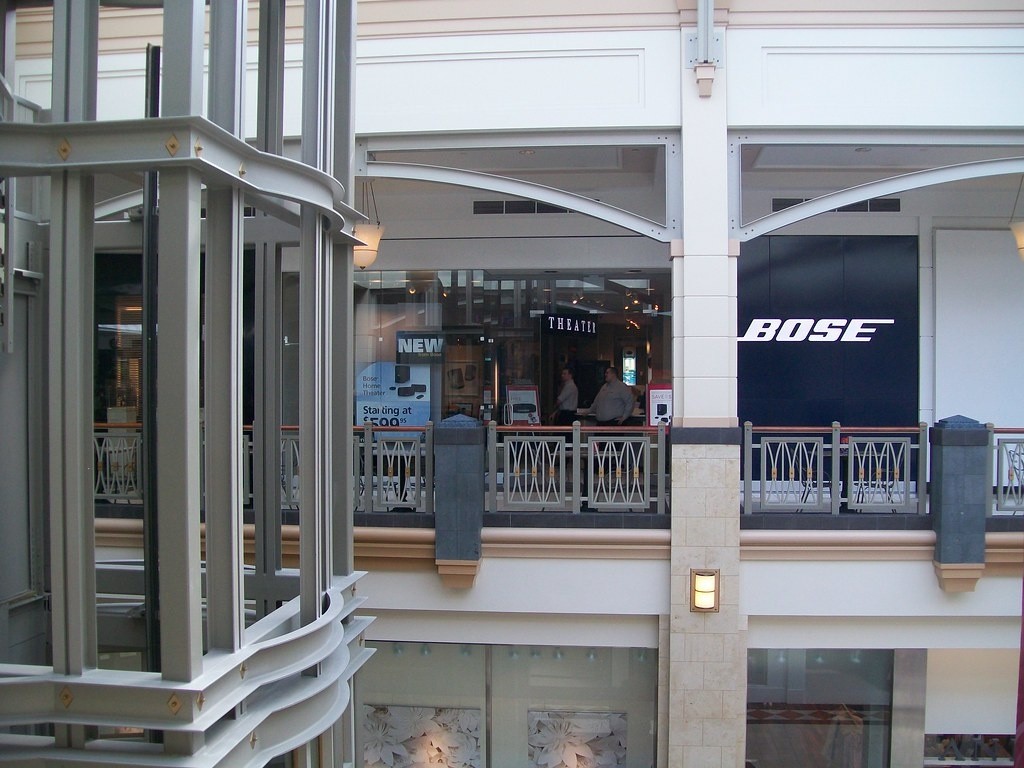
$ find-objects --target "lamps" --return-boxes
[690,568,720,612]
[346,178,385,271]
[1007,172,1024,261]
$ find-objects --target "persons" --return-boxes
[582,366,635,473]
[549,367,579,465]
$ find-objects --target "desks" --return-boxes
[806,448,888,513]
[549,450,622,514]
[362,449,426,514]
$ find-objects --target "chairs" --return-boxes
[856,441,907,514]
[787,447,834,514]
[596,438,641,504]
